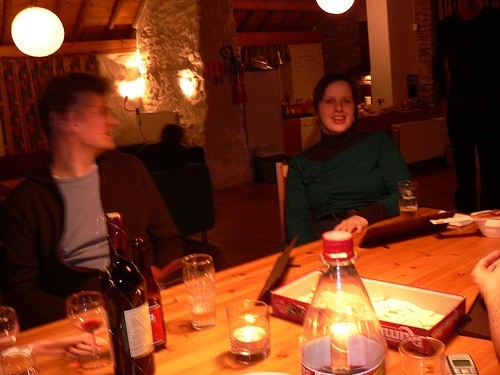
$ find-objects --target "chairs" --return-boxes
[274,161,289,248]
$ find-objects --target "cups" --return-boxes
[0,344,42,375]
[0,306,22,353]
[398,336,446,375]
[183,253,217,331]
[365,96,371,106]
[226,299,272,363]
[397,179,418,217]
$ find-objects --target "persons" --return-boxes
[158,123,185,146]
[1,72,182,333]
[432,0,500,213]
[287,72,407,247]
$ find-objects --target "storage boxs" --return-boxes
[270,268,467,356]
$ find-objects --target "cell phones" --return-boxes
[447,353,480,375]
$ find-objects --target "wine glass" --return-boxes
[66,290,112,370]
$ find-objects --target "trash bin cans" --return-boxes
[251,142,285,185]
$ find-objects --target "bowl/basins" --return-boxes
[471,211,500,239]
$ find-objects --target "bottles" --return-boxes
[299,231,390,375]
[130,235,167,352]
[101,212,156,375]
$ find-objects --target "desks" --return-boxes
[0,210,500,375]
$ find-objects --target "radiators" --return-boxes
[392,118,450,165]
